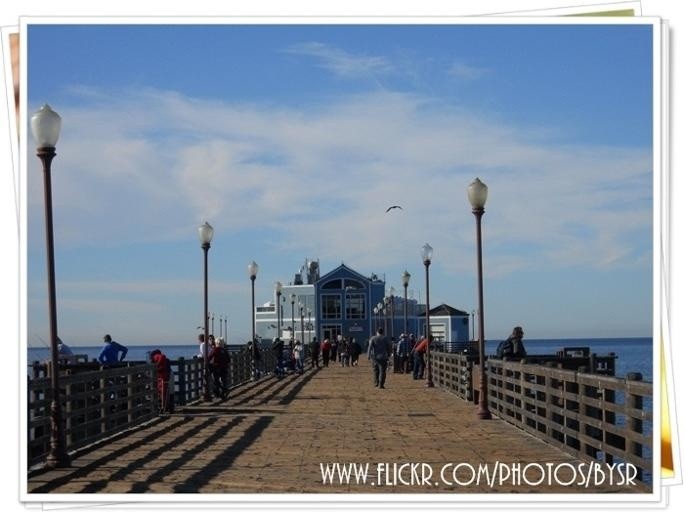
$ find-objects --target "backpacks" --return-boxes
[496,336,516,361]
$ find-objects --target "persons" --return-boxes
[391,331,433,380]
[506,326,528,362]
[149,349,171,412]
[193,332,230,402]
[97,334,128,364]
[367,328,393,388]
[57,337,75,365]
[247,331,362,379]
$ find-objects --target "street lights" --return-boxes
[461,177,496,418]
[29,104,76,466]
[286,294,313,341]
[416,241,438,390]
[373,282,394,338]
[398,266,412,334]
[190,217,220,404]
[245,258,264,381]
[273,281,282,341]
